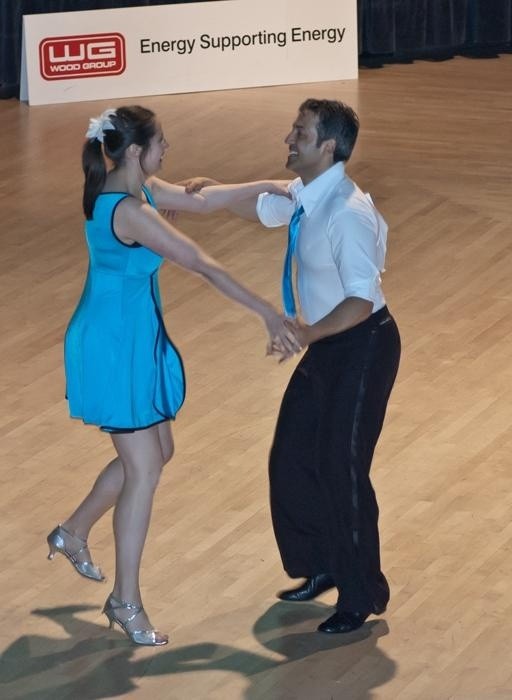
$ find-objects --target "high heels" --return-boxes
[46,524,105,581]
[104,594,167,646]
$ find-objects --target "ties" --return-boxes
[282,205,305,318]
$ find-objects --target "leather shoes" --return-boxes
[318,597,386,632]
[278,575,336,600]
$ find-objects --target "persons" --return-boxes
[46,103,304,647]
[160,99,404,635]
